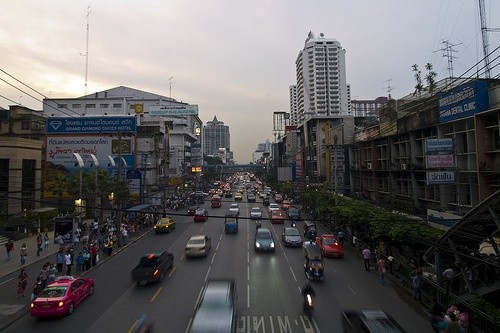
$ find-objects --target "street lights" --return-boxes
[162,144,184,211]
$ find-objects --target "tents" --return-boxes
[126,204,150,211]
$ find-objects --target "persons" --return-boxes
[5,239,14,262]
[362,245,372,271]
[338,230,345,247]
[302,279,316,298]
[377,256,386,285]
[44,233,49,250]
[36,235,42,256]
[55,205,167,276]
[30,261,57,301]
[17,267,26,297]
[456,250,495,293]
[166,193,194,211]
[20,242,27,264]
[307,239,315,247]
[413,271,422,299]
[291,220,296,227]
[430,303,469,333]
[443,265,455,294]
[255,219,261,227]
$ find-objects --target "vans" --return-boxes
[211,194,222,208]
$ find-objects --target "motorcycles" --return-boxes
[303,247,324,282]
[303,292,315,320]
[304,221,317,240]
[224,211,239,233]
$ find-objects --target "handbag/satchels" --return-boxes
[382,267,386,273]
[40,248,42,251]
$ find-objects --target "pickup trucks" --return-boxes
[131,250,174,285]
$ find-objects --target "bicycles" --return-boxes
[16,278,26,296]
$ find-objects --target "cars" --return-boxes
[29,275,95,318]
[317,233,345,259]
[188,205,209,222]
[153,217,176,234]
[341,306,410,333]
[268,200,302,224]
[255,228,276,252]
[274,193,282,201]
[229,203,241,214]
[191,190,210,197]
[185,278,238,333]
[184,234,211,259]
[250,206,262,218]
[209,171,273,206]
[281,227,302,247]
